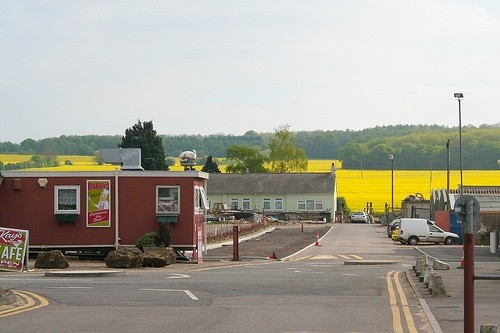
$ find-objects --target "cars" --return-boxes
[350,211,368,223]
[387,219,400,241]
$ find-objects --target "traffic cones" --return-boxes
[269,251,277,259]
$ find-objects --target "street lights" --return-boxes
[454,92,464,195]
[388,153,394,213]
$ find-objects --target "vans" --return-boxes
[398,218,459,245]
